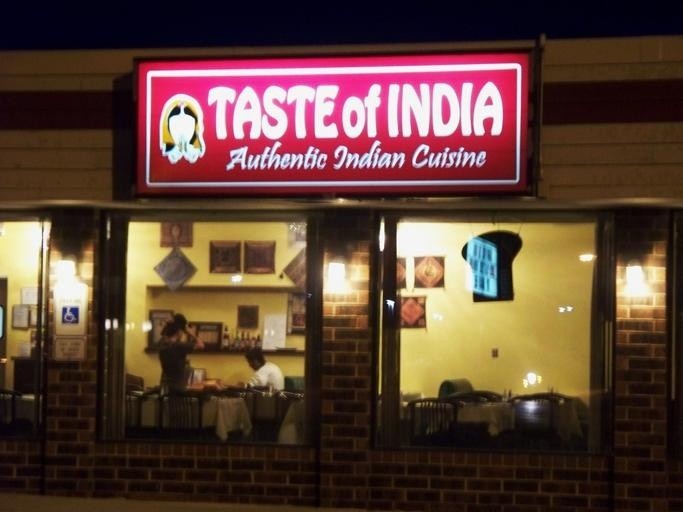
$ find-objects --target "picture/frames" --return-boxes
[244,240,276,274]
[209,240,241,273]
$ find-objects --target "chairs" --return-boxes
[0,387,33,435]
[125,374,303,440]
[404,380,570,449]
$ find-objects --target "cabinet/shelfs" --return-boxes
[144,285,305,356]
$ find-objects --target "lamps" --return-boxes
[626,258,640,267]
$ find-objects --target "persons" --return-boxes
[214,345,286,393]
[157,313,206,392]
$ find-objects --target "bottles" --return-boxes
[221,325,261,351]
[502,388,512,402]
